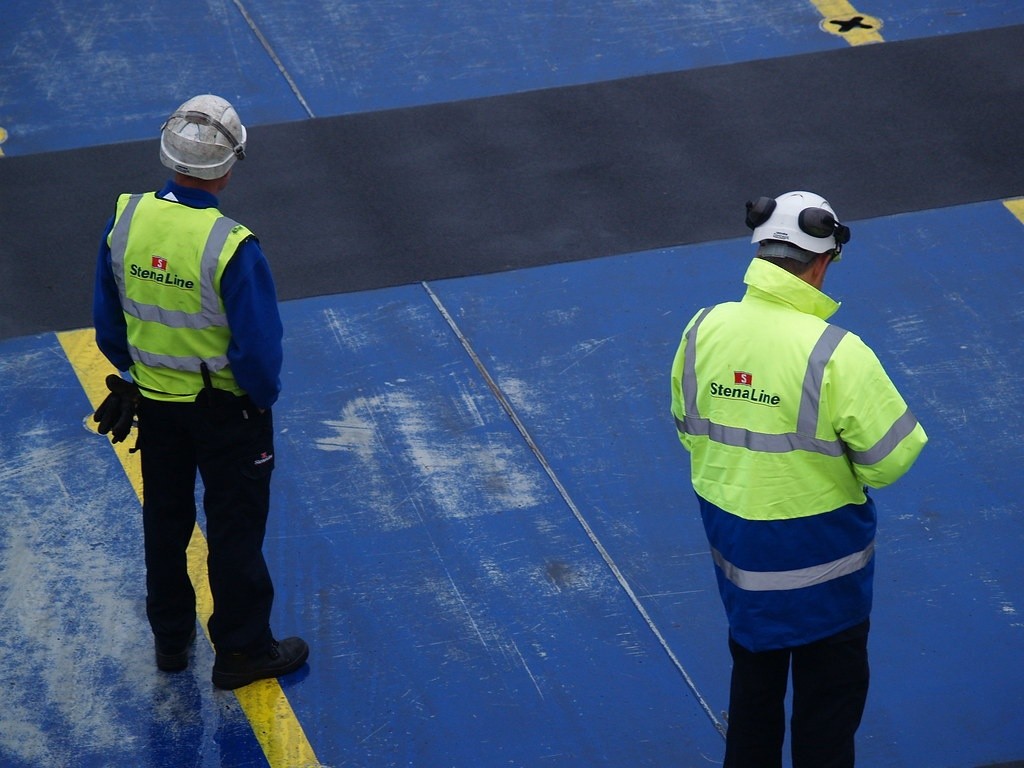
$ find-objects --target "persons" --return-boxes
[671,191,929,768]
[91,94,309,690]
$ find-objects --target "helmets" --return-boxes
[157,93,248,179]
[749,191,843,260]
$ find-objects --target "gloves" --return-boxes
[94,373,141,444]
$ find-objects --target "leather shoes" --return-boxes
[213,635,310,689]
[151,620,196,671]
